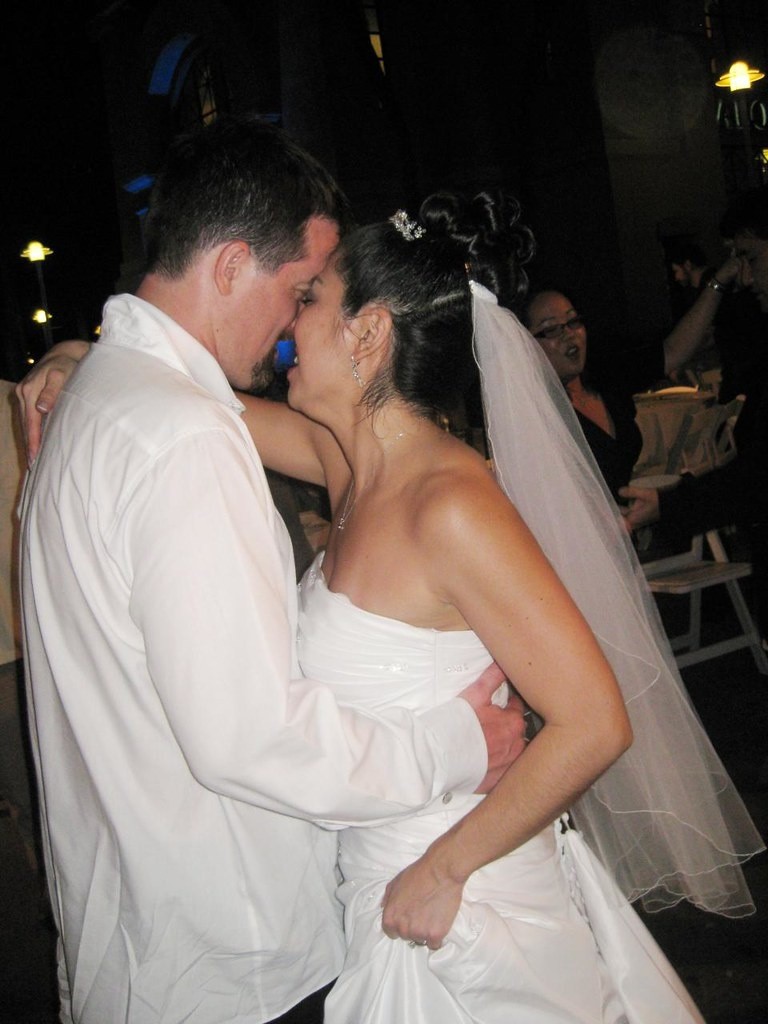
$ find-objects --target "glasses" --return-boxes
[534,313,586,339]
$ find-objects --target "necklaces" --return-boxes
[338,416,429,531]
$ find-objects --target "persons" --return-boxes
[14,190,768,1024]
[516,192,768,601]
[16,112,529,1024]
[0,376,44,887]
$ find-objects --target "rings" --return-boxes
[408,940,426,947]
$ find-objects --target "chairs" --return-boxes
[630,383,767,678]
